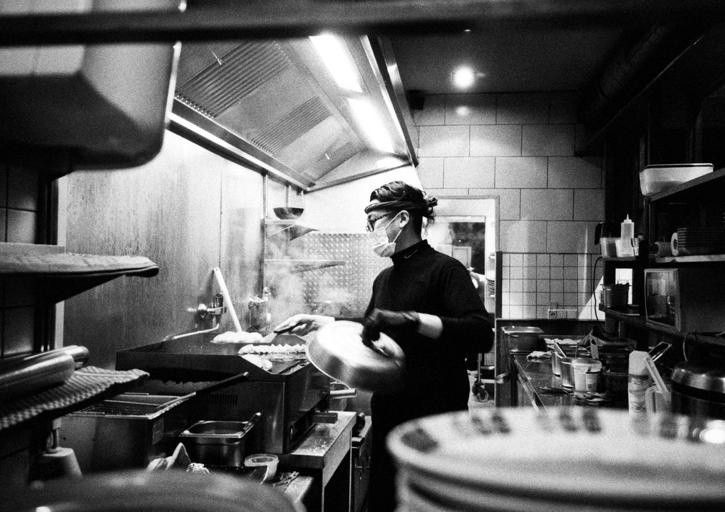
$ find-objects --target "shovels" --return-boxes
[260,325,293,344]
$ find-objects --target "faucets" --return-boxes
[198,291,217,319]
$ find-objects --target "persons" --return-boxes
[273,181,494,512]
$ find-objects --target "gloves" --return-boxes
[361,307,421,342]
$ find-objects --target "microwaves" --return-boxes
[644,267,724,334]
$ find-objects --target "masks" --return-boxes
[370,212,403,258]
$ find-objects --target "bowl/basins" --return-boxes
[560,356,602,388]
[273,207,303,220]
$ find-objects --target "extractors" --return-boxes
[171,23,417,193]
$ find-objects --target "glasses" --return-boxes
[366,213,392,232]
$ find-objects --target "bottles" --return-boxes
[627,350,652,412]
[620,214,634,256]
